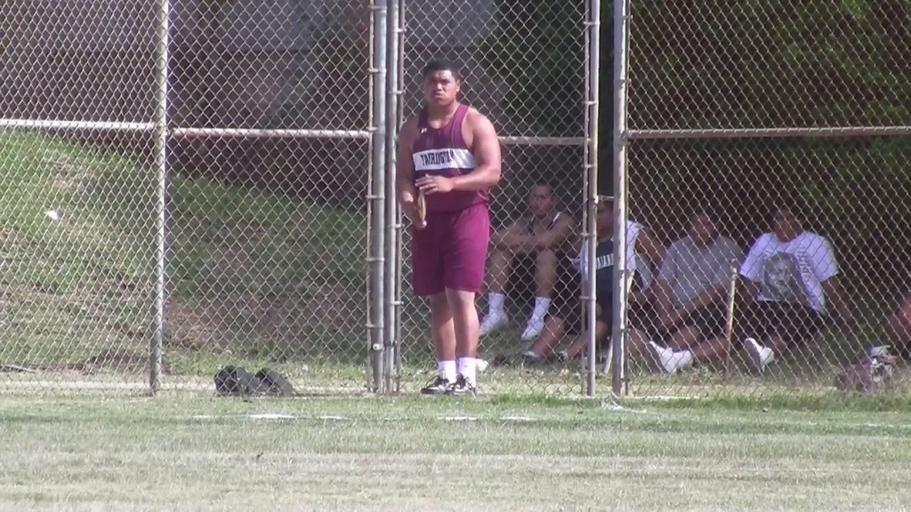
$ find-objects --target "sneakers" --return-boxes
[647,341,676,377]
[744,336,764,375]
[520,318,545,341]
[478,312,509,337]
[443,377,478,397]
[420,376,456,394]
[494,351,576,368]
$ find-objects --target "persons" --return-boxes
[479,175,580,344]
[508,188,667,366]
[625,207,747,374]
[647,203,859,378]
[393,61,503,397]
